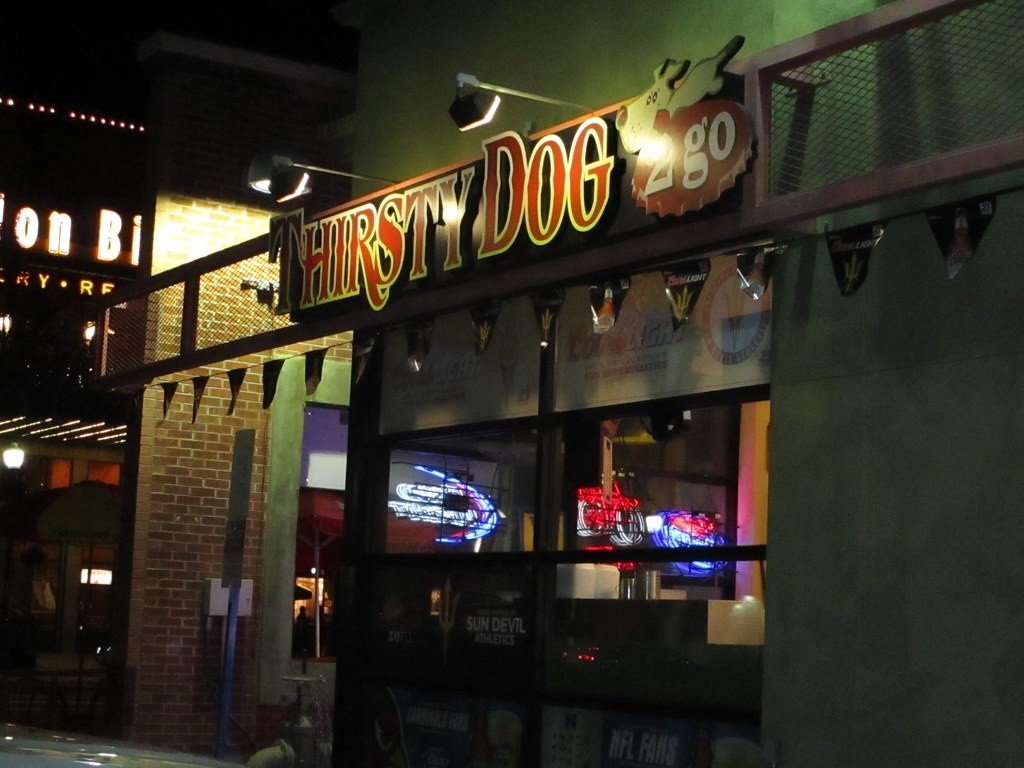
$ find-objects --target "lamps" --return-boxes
[448,73,595,133]
[246,154,398,204]
